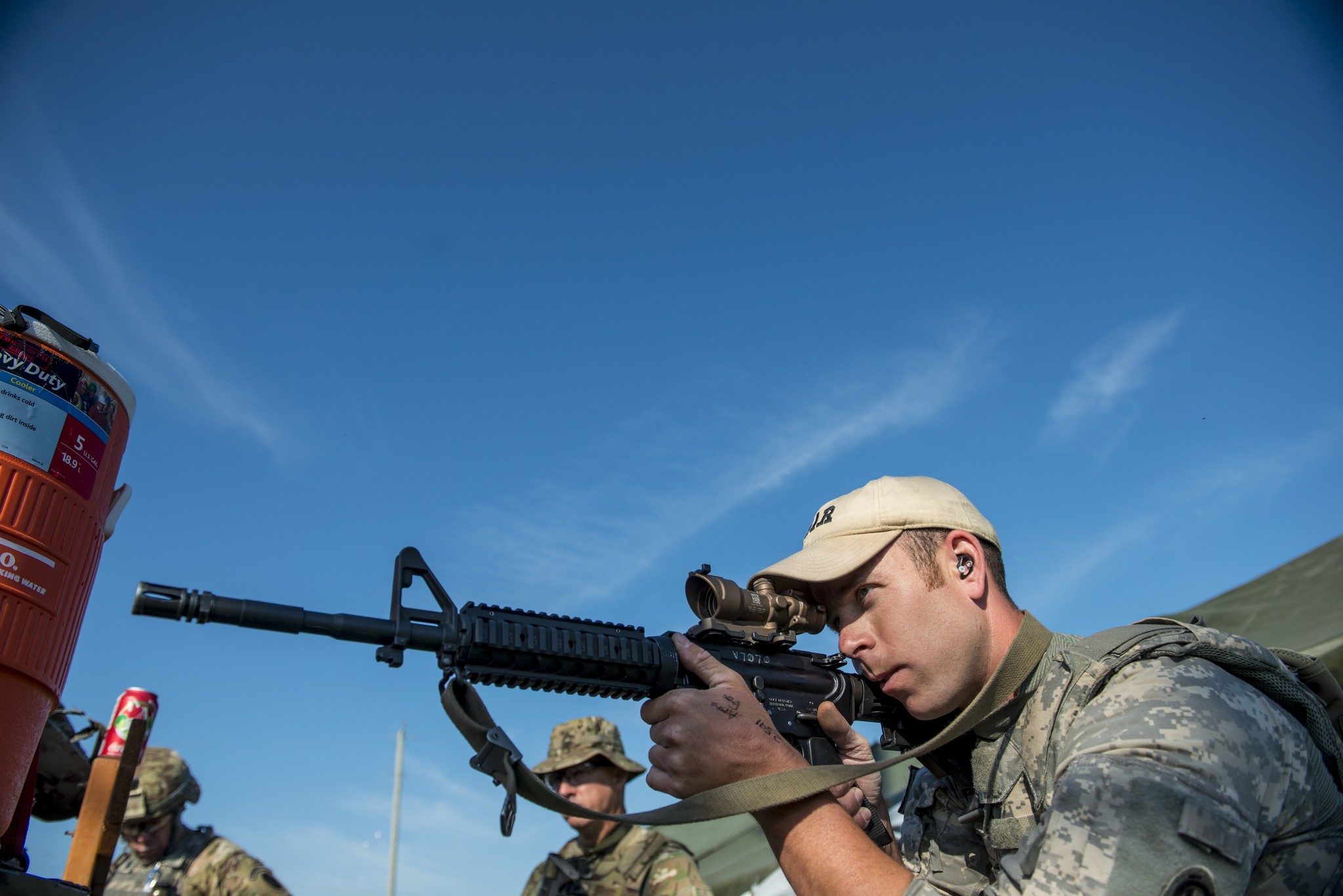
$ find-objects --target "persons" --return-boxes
[513,717,709,896]
[645,475,1343,896]
[97,745,290,896]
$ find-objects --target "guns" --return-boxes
[130,543,958,853]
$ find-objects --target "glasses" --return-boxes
[548,755,608,788]
[118,808,181,836]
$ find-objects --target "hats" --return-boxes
[747,472,1004,592]
[119,747,202,823]
[530,716,645,783]
